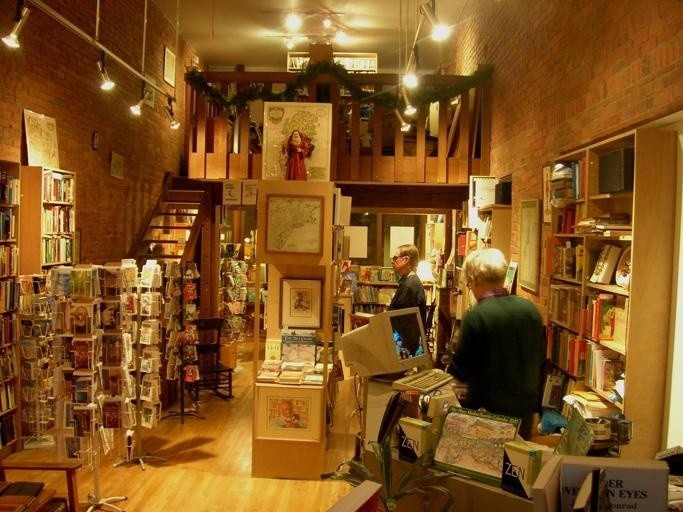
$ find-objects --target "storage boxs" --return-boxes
[220,336,237,371]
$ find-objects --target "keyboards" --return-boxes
[392,368,454,394]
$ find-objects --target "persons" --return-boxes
[447,249,546,443]
[281,130,309,180]
[387,243,427,354]
[268,402,306,429]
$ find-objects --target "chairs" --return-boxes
[187,316,234,408]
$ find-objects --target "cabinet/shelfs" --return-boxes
[154,221,265,333]
[286,51,377,118]
[333,204,511,368]
[0,159,76,457]
[545,130,676,460]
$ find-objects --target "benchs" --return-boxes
[0,462,83,512]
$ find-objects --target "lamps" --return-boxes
[395,0,450,133]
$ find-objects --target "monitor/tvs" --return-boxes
[341,307,430,382]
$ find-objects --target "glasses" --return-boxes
[393,256,405,261]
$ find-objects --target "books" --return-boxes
[547,164,632,421]
[427,175,499,351]
[43,169,74,273]
[219,240,267,334]
[351,265,433,329]
[1,172,20,413]
[0,477,64,512]
[256,338,333,386]
[334,188,352,338]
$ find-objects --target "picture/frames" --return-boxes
[520,199,540,294]
[143,71,156,108]
[278,276,322,329]
[265,193,324,254]
[254,383,325,443]
[163,45,176,88]
[0,0,181,130]
[262,100,333,182]
[542,165,551,225]
